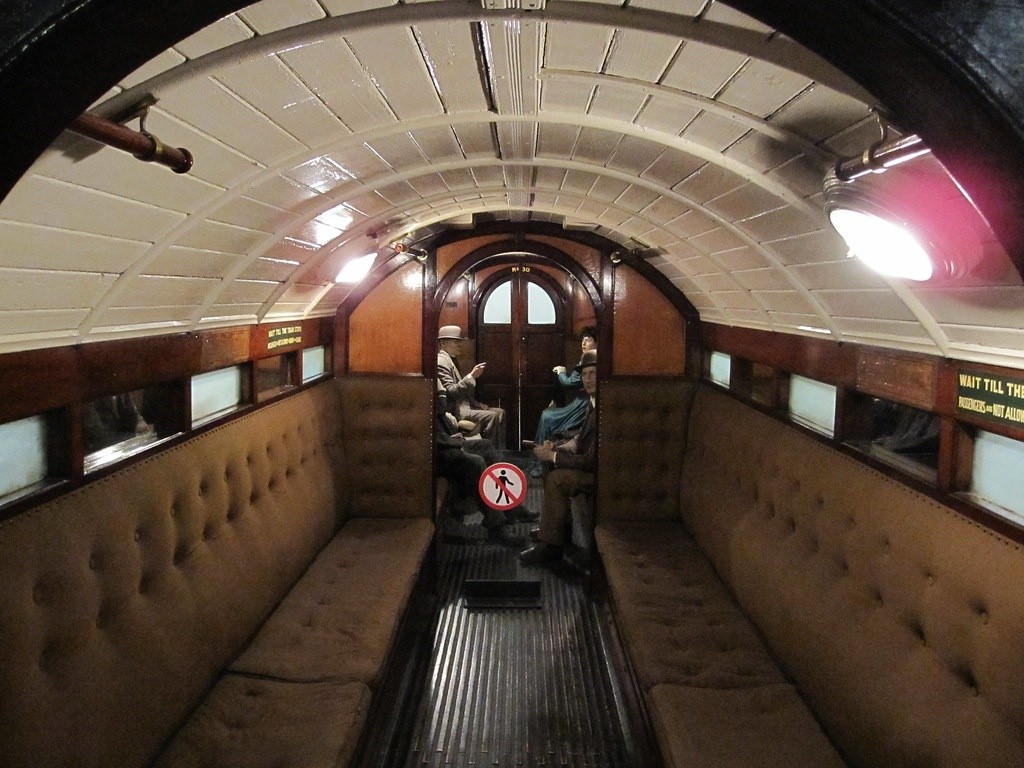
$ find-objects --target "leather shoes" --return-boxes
[519,544,561,562]
[530,527,541,537]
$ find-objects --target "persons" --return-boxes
[519,327,596,563]
[437,325,541,532]
[82,390,151,452]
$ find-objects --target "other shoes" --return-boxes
[506,508,540,523]
[487,528,524,545]
[530,463,542,478]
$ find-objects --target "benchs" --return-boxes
[0,386,1024,768]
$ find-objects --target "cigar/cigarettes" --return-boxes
[476,366,480,369]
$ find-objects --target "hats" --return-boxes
[437,325,467,340]
[574,349,597,375]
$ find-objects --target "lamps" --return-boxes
[822,168,987,288]
